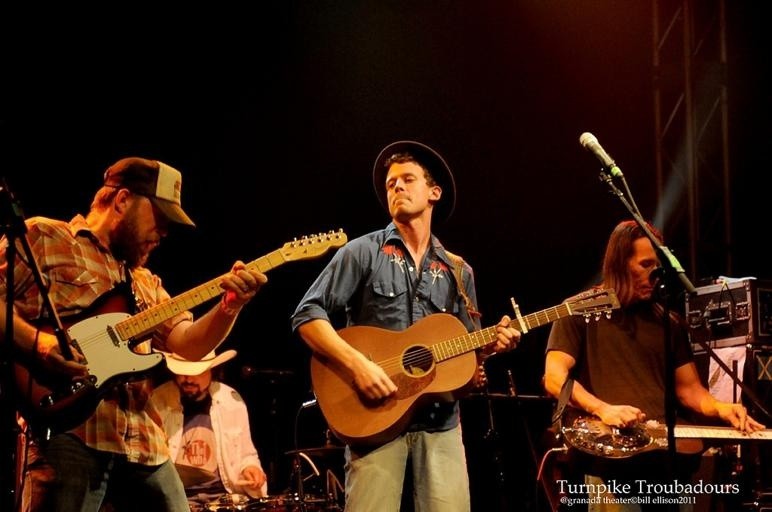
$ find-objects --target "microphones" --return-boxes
[579,132,624,178]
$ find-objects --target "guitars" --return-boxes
[1,228,347,429]
[309,285,621,446]
[560,406,772,460]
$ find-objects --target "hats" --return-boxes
[372,140,455,222]
[152,347,238,376]
[104,156,197,229]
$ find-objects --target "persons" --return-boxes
[149,345,271,512]
[541,218,766,512]
[291,140,522,512]
[0,157,269,512]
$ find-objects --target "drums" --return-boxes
[187,493,330,511]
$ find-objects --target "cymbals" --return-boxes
[174,462,220,488]
[285,445,346,458]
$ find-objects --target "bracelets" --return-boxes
[221,297,241,316]
